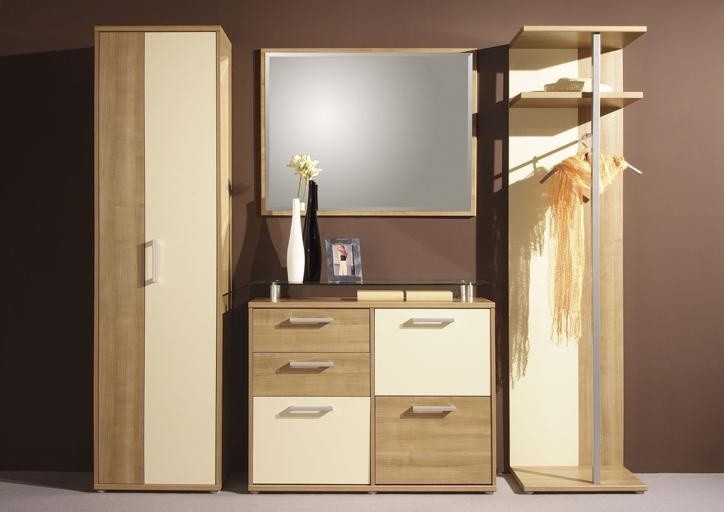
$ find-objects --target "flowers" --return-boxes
[303,160,323,202]
[287,153,311,198]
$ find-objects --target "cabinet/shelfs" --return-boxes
[93,24,235,494]
[246,295,498,495]
[504,24,648,495]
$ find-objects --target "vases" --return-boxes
[286,198,305,284]
[300,202,305,211]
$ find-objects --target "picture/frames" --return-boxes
[324,238,363,286]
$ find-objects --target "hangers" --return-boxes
[538,133,643,184]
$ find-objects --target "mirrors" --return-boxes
[260,48,478,218]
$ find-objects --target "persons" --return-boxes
[337,245,348,275]
